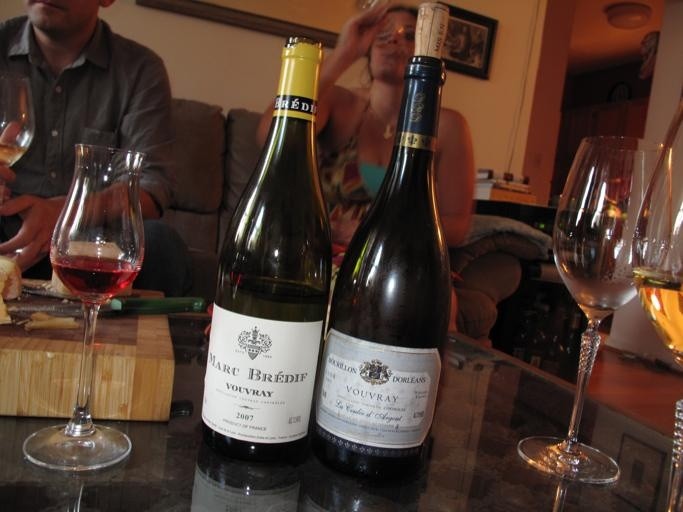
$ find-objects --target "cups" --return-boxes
[0,70,37,170]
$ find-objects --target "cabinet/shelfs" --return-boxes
[473,195,607,350]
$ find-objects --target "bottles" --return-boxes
[307,0,451,511]
[200,38,333,491]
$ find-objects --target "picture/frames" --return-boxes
[436,2,500,82]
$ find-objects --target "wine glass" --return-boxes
[22,144,146,470]
[631,112,682,512]
[517,136,675,485]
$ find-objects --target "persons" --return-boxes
[0,1,193,329]
[253,0,475,251]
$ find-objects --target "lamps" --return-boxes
[603,3,652,31]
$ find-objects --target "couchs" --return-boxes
[120,98,551,351]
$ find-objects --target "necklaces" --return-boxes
[370,102,399,139]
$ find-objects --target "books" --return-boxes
[472,178,537,208]
